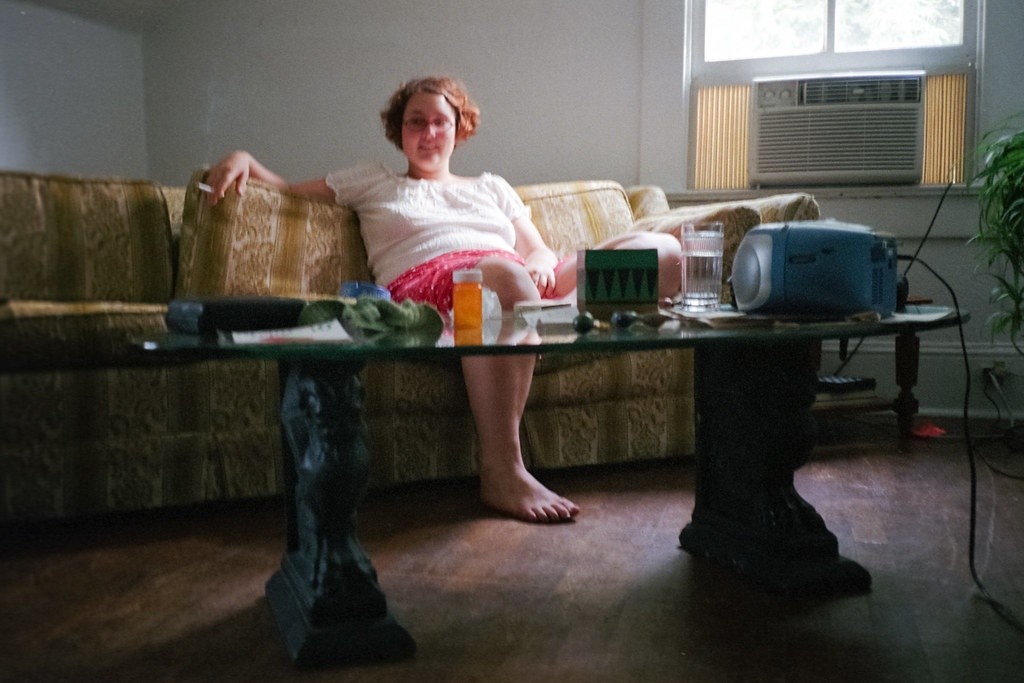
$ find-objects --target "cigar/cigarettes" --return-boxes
[195,181,215,194]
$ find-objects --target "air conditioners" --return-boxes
[747,66,978,187]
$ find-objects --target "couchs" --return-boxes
[0,184,826,533]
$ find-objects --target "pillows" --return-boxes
[514,180,636,263]
[173,163,375,303]
[0,168,173,302]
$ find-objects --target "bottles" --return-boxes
[453,270,483,328]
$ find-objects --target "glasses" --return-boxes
[403,118,455,132]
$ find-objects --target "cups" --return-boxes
[681,221,724,311]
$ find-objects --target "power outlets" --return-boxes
[974,363,1007,387]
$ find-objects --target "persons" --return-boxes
[206,76,683,524]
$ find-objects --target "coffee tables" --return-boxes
[139,299,969,668]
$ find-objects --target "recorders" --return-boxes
[731,220,898,321]
